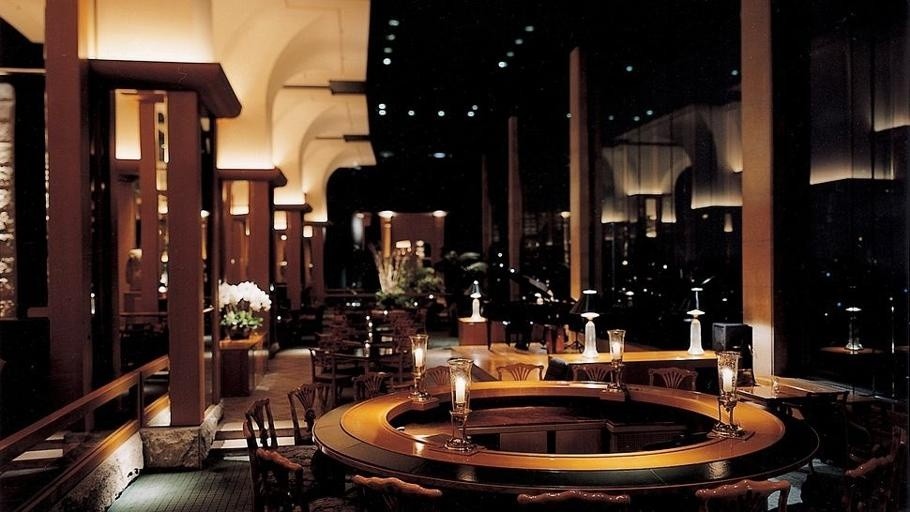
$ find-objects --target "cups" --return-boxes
[448,357,473,411]
[607,330,626,367]
[717,351,739,399]
[409,333,428,376]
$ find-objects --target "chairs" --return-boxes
[306,277,731,413]
[350,475,444,511]
[242,399,340,509]
[250,450,345,510]
[780,390,908,509]
[516,491,632,510]
[694,478,792,511]
[288,383,336,446]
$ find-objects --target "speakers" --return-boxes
[712,322,748,352]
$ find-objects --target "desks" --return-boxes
[313,379,819,504]
[732,384,850,411]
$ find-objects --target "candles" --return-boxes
[722,366,735,393]
[612,341,622,358]
[455,374,466,404]
[413,345,424,367]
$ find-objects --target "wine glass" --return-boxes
[581,312,600,358]
[686,310,706,355]
